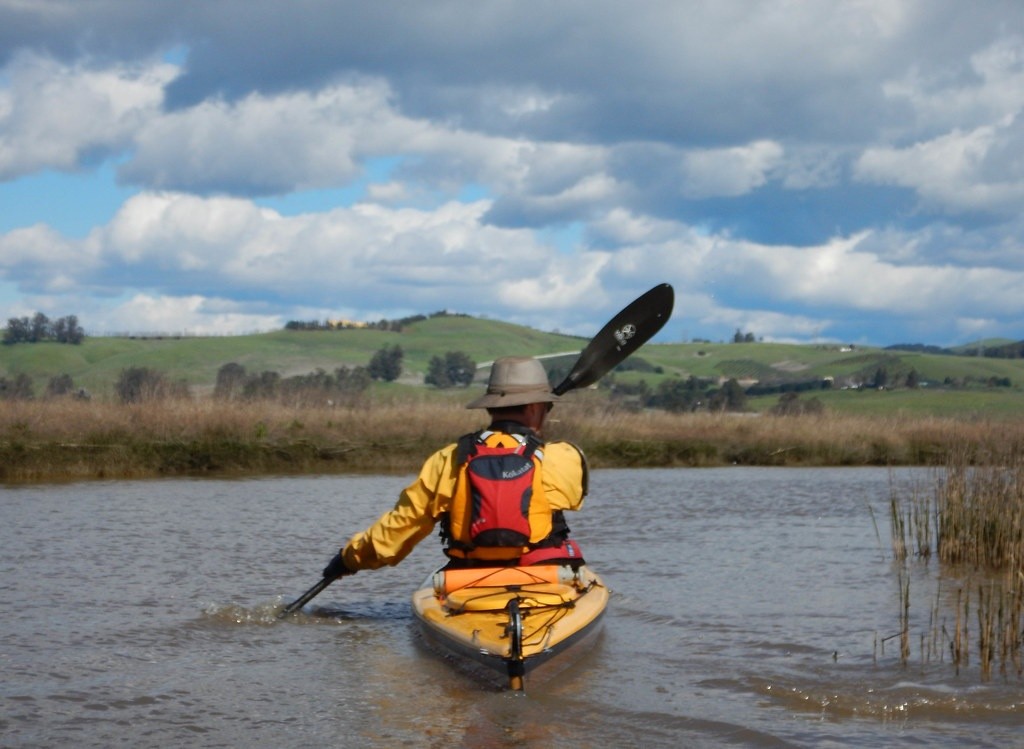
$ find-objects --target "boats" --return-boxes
[410,561,610,691]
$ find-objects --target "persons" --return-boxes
[322,360,588,582]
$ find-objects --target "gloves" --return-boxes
[322,548,357,580]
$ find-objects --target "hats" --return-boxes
[466,356,568,410]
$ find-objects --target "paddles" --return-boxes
[277,284,674,628]
